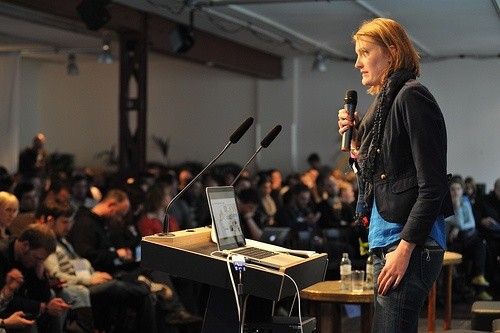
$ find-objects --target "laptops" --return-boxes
[259,227,290,245]
[205,186,304,269]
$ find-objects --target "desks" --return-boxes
[428,252,463,333]
[300,280,375,333]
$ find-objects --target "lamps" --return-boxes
[310,50,329,75]
[65,36,115,78]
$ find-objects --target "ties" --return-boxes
[57,238,76,259]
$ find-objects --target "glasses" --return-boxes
[60,218,75,225]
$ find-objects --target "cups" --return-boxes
[351,270,364,293]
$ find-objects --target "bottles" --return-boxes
[340,253,352,288]
[366,253,373,282]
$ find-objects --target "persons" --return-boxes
[0,128,500,333]
[338,18,454,333]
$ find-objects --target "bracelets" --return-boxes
[352,145,360,151]
[247,216,252,220]
[1,319,6,328]
[44,304,48,313]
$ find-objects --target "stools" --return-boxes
[471,300,500,333]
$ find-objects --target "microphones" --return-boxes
[341,90,357,152]
[162,117,254,234]
[231,124,282,186]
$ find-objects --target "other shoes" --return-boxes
[168,310,202,324]
[477,291,493,301]
[472,274,489,286]
